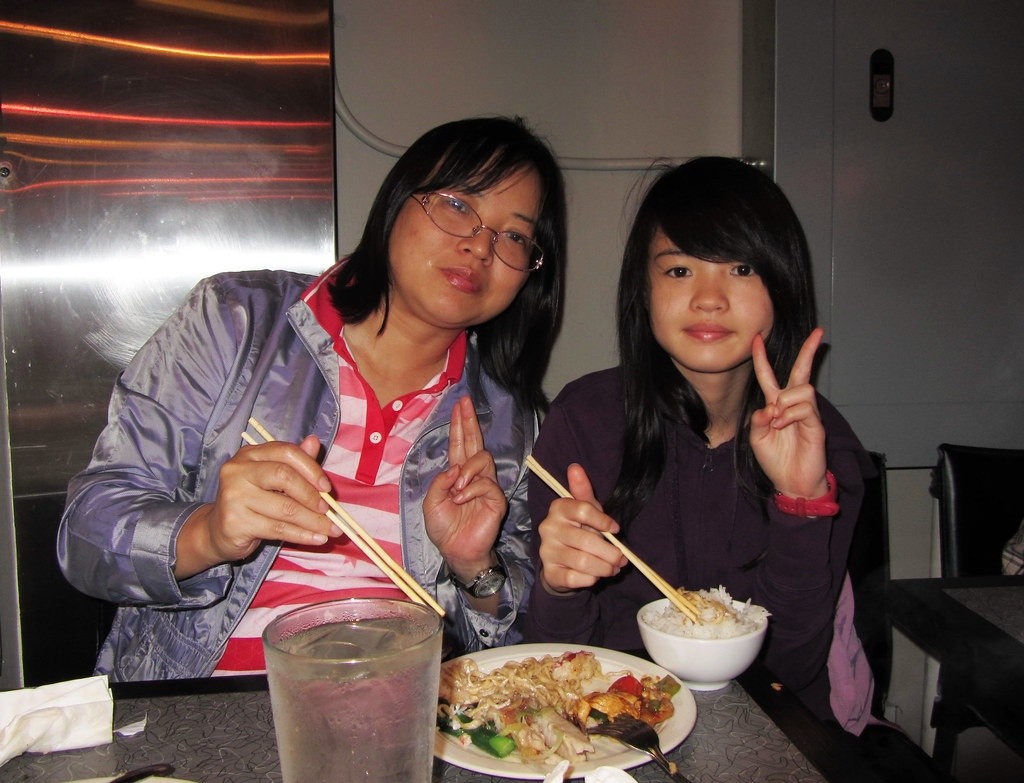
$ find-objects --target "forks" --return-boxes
[589,717,693,782]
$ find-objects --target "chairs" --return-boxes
[932,443,1023,776]
[849,447,896,727]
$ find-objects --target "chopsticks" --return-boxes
[241,418,448,619]
[525,454,699,625]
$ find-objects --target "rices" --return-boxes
[641,585,772,639]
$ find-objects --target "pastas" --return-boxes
[431,653,631,729]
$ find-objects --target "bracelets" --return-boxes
[772,470,839,519]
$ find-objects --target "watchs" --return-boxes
[443,561,507,600]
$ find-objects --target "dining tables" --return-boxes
[0,646,886,783]
[889,571,1024,755]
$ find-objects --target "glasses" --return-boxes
[410,185,545,271]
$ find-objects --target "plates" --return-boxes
[429,642,697,781]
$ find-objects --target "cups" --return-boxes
[263,599,444,783]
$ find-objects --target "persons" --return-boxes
[56,114,572,689]
[525,154,928,782]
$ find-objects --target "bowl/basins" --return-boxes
[637,598,769,692]
